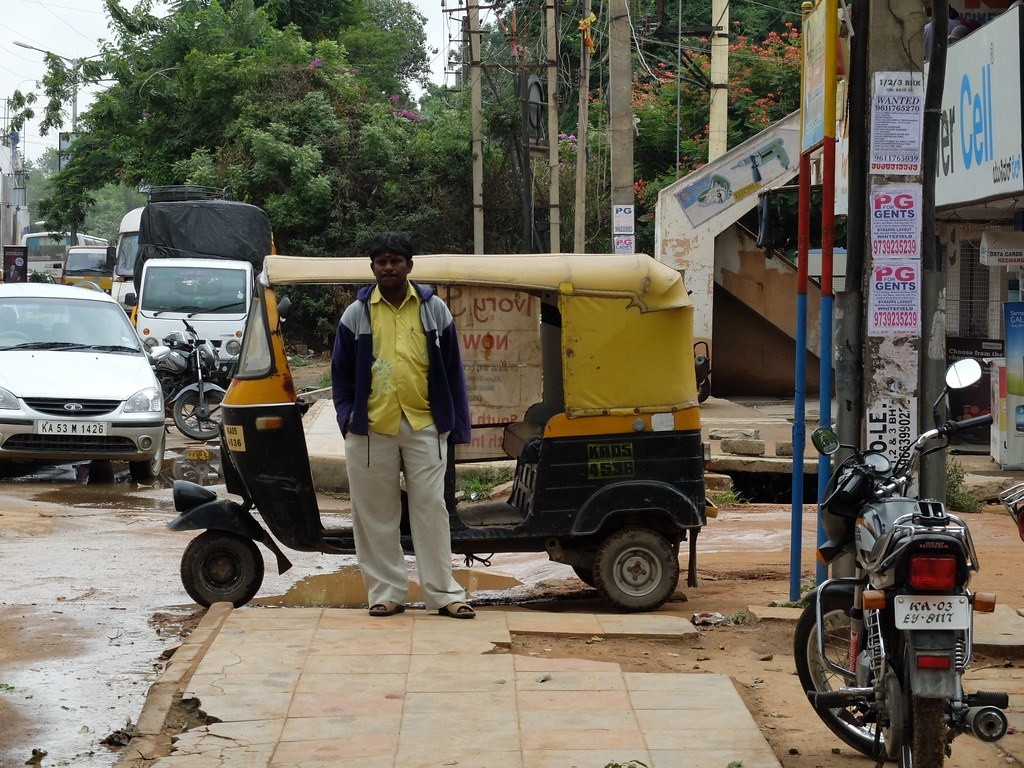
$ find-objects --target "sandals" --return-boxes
[439,602,476,618]
[370,599,405,616]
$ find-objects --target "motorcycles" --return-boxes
[794,356,1009,768]
[167,252,716,609]
[143,318,230,441]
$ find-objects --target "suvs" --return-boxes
[0,281,166,482]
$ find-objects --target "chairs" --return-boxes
[0,306,18,338]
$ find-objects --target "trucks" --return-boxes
[106,204,147,317]
[125,199,280,336]
[52,246,117,294]
[124,260,255,370]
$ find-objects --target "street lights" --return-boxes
[14,40,124,246]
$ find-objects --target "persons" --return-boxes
[328,230,476,619]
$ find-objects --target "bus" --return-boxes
[21,232,110,285]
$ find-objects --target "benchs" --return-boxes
[501,421,543,464]
